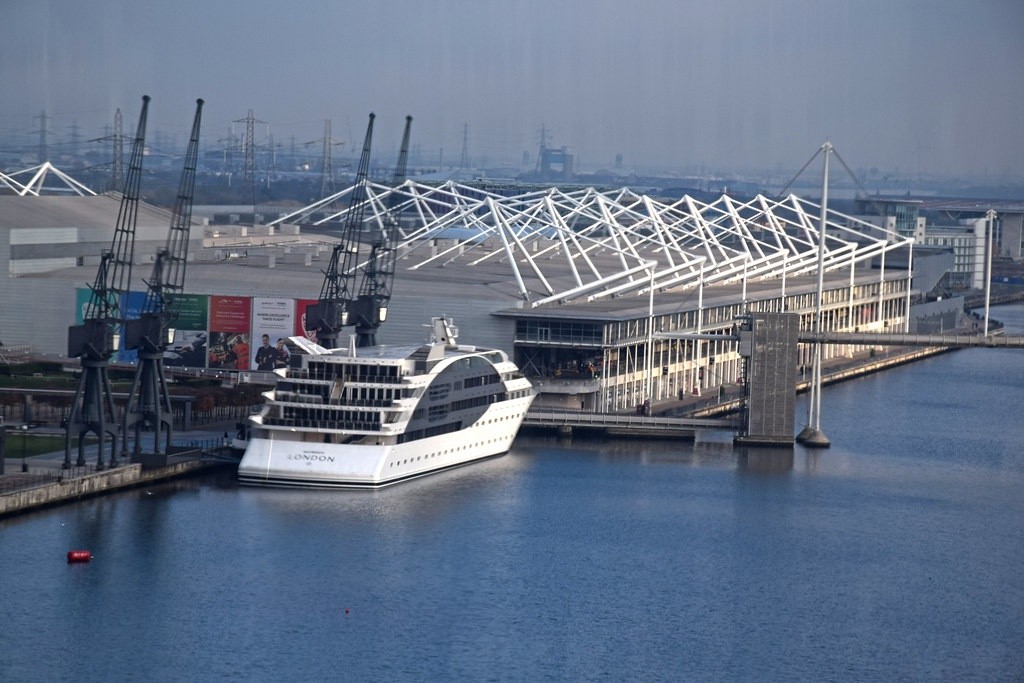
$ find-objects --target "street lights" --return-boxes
[22,424,28,472]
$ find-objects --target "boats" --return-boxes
[238,313,539,489]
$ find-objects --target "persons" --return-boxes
[255,334,277,370]
[179,333,206,355]
[230,336,249,370]
[273,338,290,369]
